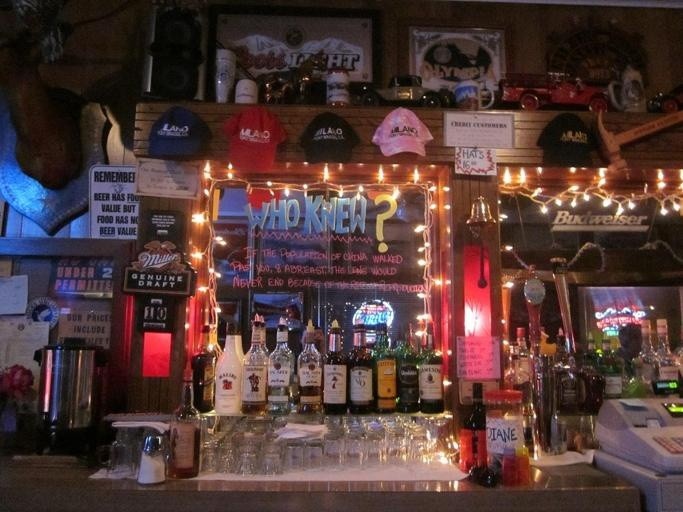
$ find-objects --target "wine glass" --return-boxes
[105,421,134,479]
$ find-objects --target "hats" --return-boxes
[371,108,434,157]
[536,113,594,166]
[300,112,359,163]
[222,107,286,172]
[148,107,210,155]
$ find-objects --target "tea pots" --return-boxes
[608,68,647,113]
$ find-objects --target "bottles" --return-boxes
[192,314,445,418]
[134,435,166,486]
[630,317,683,385]
[166,383,204,480]
[505,326,566,458]
[455,381,487,474]
[486,389,523,471]
[586,328,625,398]
[215,51,351,105]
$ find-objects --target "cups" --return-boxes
[284,437,323,473]
[202,419,280,479]
[322,419,451,468]
[452,80,493,110]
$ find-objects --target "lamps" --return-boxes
[465,197,502,287]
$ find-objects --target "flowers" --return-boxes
[2,363,37,406]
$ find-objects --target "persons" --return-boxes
[613,320,643,374]
[283,303,304,330]
